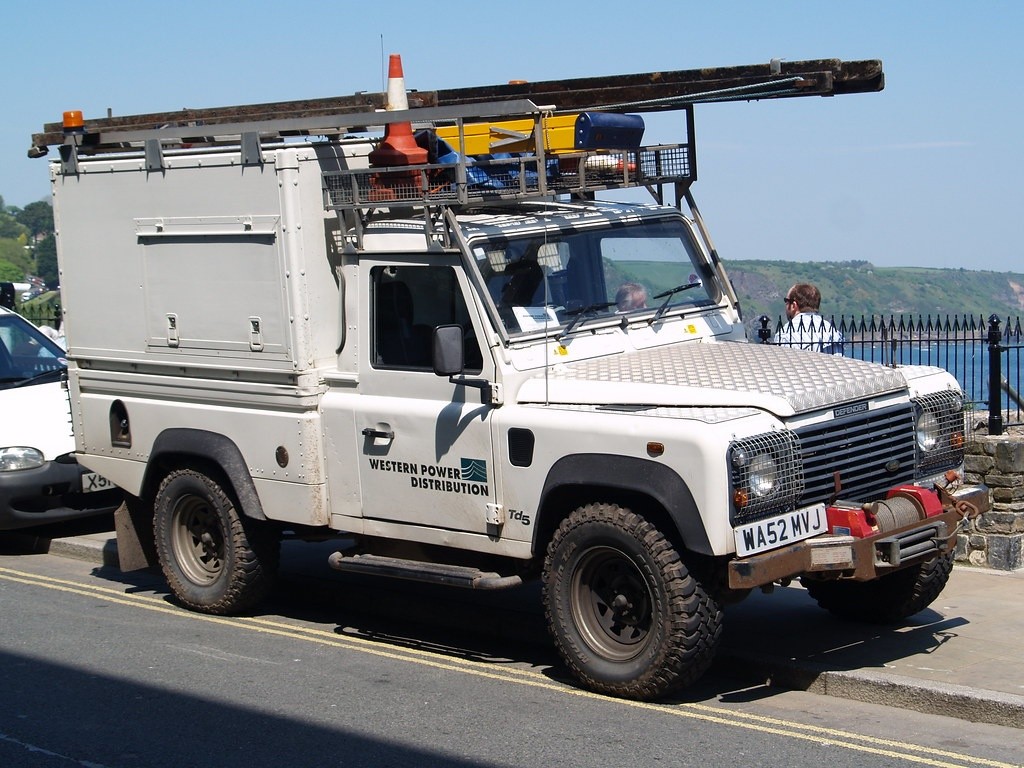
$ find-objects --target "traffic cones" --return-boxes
[585,156,636,173]
[368,53,429,200]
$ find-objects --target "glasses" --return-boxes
[784,297,794,304]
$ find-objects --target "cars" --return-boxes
[0,305,124,532]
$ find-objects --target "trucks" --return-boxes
[50,137,990,703]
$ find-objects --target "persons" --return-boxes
[615,283,646,314]
[773,282,845,357]
